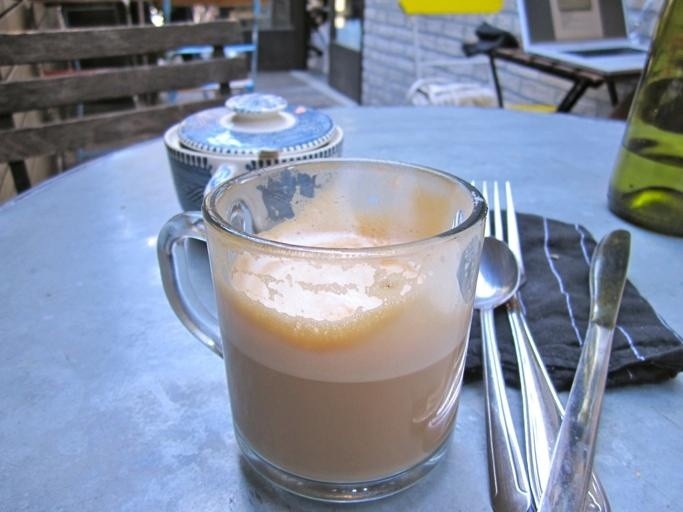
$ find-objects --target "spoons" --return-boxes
[470,238,532,508]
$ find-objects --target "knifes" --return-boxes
[541,229,632,507]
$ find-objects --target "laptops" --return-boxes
[515,0,649,78]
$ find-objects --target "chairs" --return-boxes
[0,18,253,196]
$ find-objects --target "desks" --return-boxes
[0,106,681,511]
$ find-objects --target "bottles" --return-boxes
[609,2,683,242]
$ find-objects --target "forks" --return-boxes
[471,177,615,508]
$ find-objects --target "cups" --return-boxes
[156,155,489,505]
[157,91,347,215]
